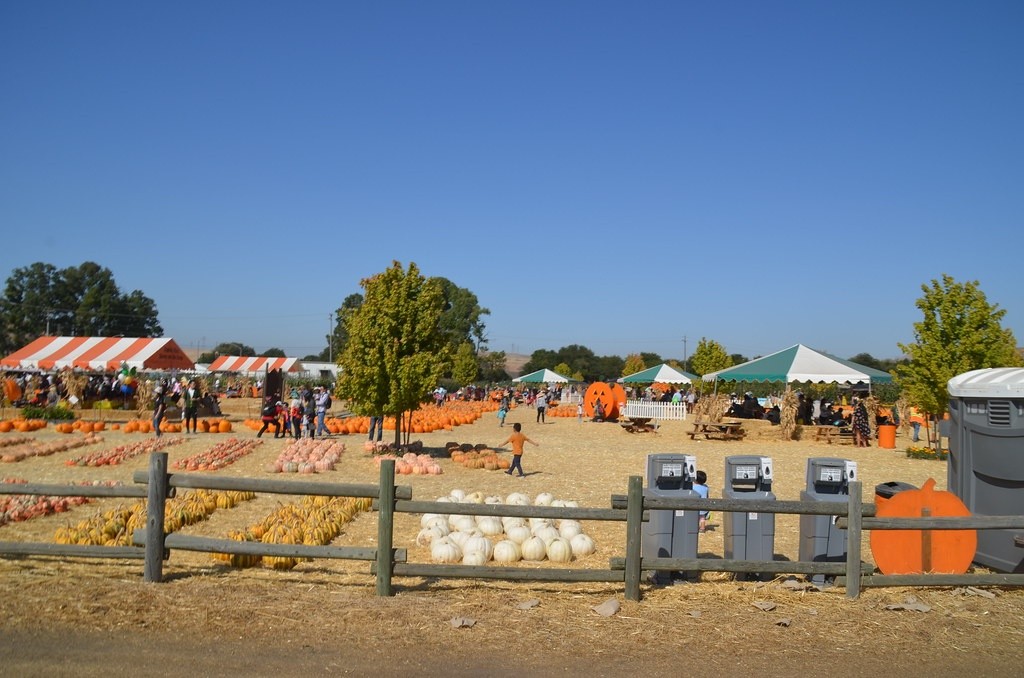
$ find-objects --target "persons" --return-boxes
[1,362,385,443]
[692,471,711,533]
[497,422,539,478]
[427,381,925,448]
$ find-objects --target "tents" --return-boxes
[617,363,704,414]
[1,335,199,412]
[207,355,310,405]
[700,343,896,444]
[512,369,579,407]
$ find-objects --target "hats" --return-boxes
[154,386,162,395]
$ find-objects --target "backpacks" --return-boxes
[263,404,276,416]
[322,392,332,409]
[598,404,605,414]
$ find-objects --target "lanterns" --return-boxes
[584,378,615,419]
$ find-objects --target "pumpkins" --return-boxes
[1,393,598,570]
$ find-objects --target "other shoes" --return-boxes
[503,471,513,476]
[498,424,503,427]
[541,422,545,425]
[154,431,164,439]
[515,474,525,479]
[537,421,540,424]
[698,528,706,533]
[596,418,604,422]
[192,430,199,435]
[184,430,189,435]
[255,431,331,442]
